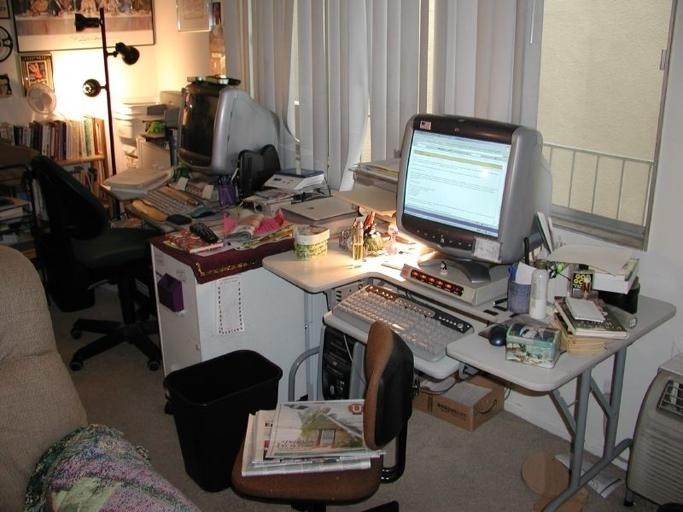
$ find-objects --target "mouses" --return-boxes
[489,326,506,346]
[191,206,217,218]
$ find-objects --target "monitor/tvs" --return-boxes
[174,83,280,200]
[396,113,553,306]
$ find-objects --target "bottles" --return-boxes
[572,265,593,298]
[352,222,364,266]
[528,260,549,319]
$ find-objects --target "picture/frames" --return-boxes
[19,53,55,98]
[211,1,221,27]
[12,0,157,53]
[177,0,211,34]
[0,0,11,19]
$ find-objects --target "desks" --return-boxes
[262,233,676,512]
[99,167,327,404]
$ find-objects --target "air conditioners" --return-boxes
[624,352,683,508]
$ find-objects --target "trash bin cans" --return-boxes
[162,349,283,493]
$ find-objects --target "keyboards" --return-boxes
[332,283,475,363]
[140,188,205,216]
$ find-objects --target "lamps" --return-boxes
[74,7,139,220]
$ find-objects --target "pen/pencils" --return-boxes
[217,167,239,185]
[523,236,530,265]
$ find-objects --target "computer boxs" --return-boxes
[317,325,367,401]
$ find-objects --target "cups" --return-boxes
[507,280,531,314]
[219,184,235,206]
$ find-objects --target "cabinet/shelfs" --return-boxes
[0,117,113,305]
[135,138,172,171]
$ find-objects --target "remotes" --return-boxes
[189,223,219,244]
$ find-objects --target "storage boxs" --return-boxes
[429,375,505,432]
[411,374,459,413]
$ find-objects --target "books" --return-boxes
[1,112,109,252]
[164,230,224,256]
[550,296,630,352]
[588,257,641,295]
[278,197,360,241]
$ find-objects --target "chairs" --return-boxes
[31,156,162,370]
[0,246,196,512]
[230,320,414,512]
